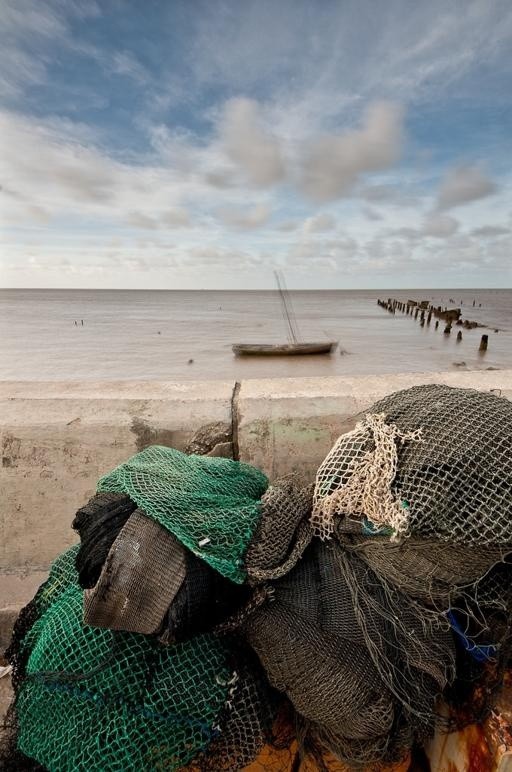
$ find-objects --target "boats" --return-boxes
[231,341,337,357]
